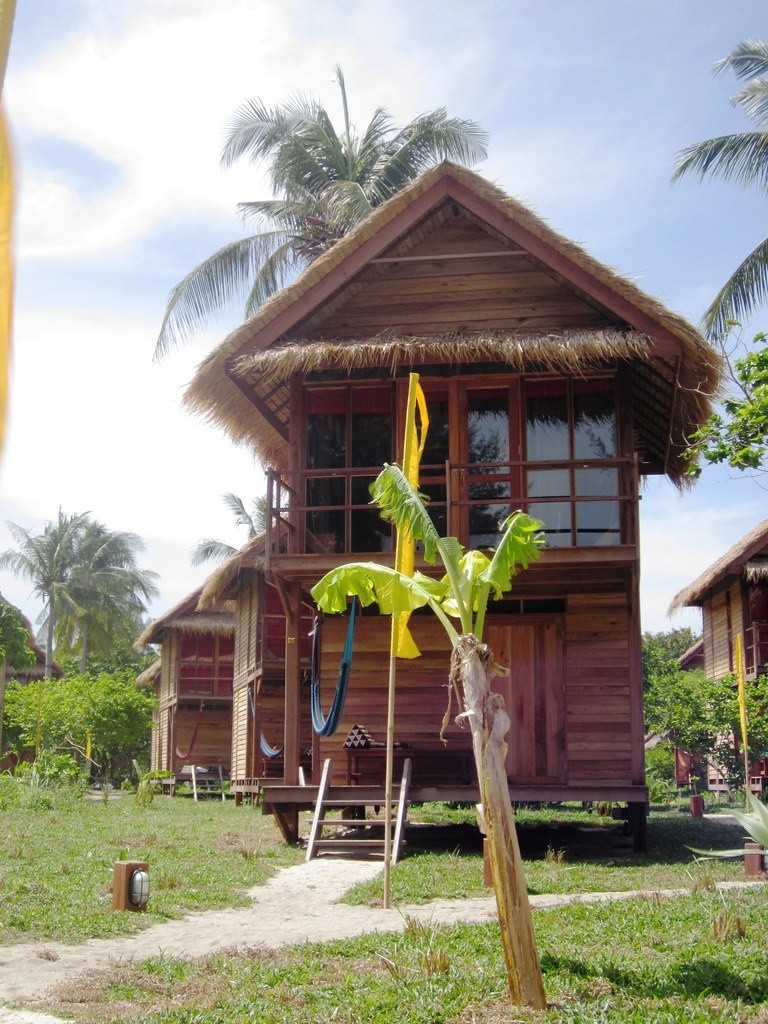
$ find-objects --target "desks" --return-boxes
[261,757,312,778]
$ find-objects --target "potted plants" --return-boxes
[689,775,704,818]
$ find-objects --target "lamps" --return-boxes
[112,860,149,915]
